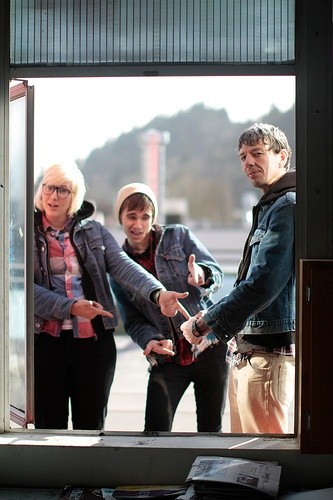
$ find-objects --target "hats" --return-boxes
[114,183,158,228]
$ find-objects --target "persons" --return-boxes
[105,181,228,433]
[31,160,192,431]
[177,121,296,434]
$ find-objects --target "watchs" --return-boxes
[191,320,206,339]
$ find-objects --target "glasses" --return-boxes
[42,183,72,199]
